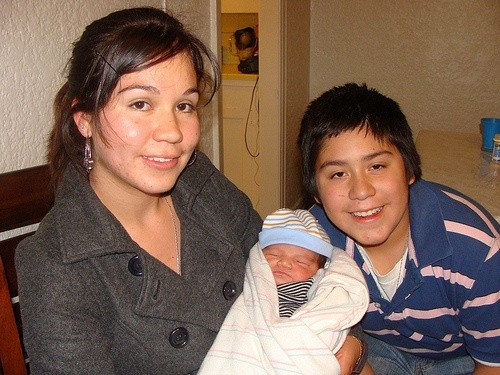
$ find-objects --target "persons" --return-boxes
[297,82,500,375]
[196,207,370,375]
[14,6,265,375]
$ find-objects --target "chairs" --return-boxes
[0,162,55,375]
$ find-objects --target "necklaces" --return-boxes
[361,233,408,301]
[164,195,181,274]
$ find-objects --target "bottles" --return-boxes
[491,133,500,160]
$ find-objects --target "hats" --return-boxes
[258,208,333,259]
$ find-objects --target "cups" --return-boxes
[480,118,500,152]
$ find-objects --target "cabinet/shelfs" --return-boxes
[221,64,259,212]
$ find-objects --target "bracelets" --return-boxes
[352,336,363,365]
